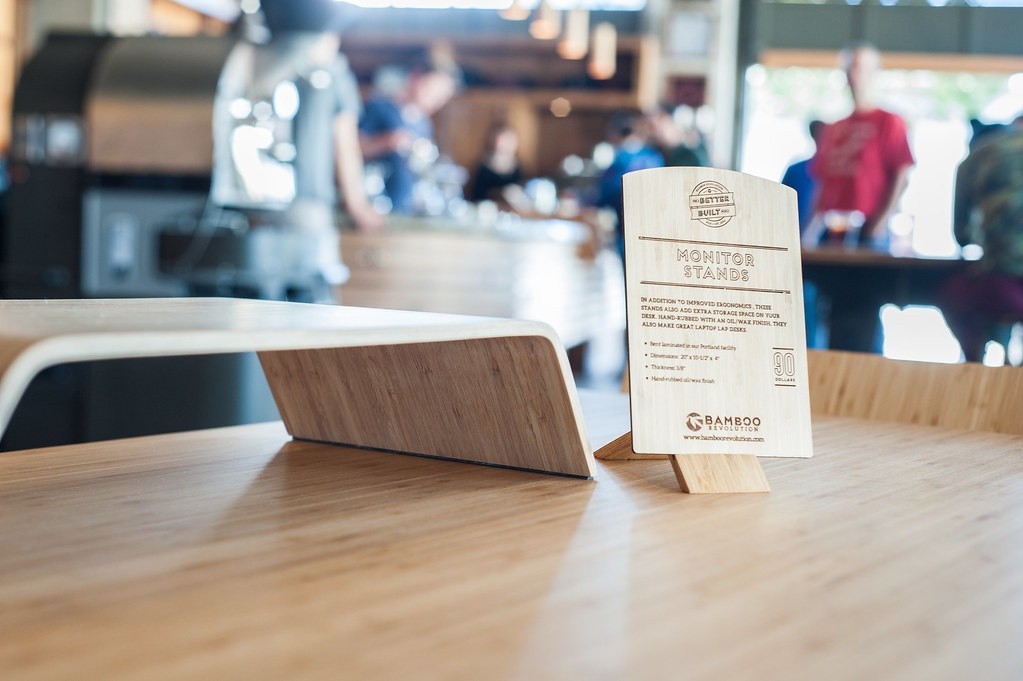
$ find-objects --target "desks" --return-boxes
[0,393,1023,681]
[802,250,1022,364]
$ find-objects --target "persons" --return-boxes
[411,39,491,90]
[272,31,383,306]
[940,115,1023,366]
[359,50,464,218]
[953,118,1009,366]
[808,43,916,354]
[558,99,714,394]
[463,121,532,215]
[781,121,833,352]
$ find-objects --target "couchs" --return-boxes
[335,216,628,387]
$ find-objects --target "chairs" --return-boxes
[806,348,1022,435]
